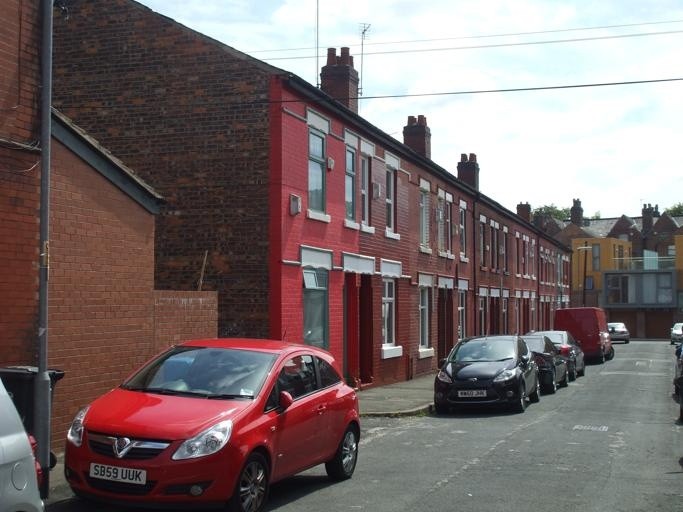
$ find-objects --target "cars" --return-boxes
[430,306,632,416]
[62,336,362,511]
[669,321,682,397]
[0,375,50,510]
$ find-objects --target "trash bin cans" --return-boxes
[0,366,64,468]
